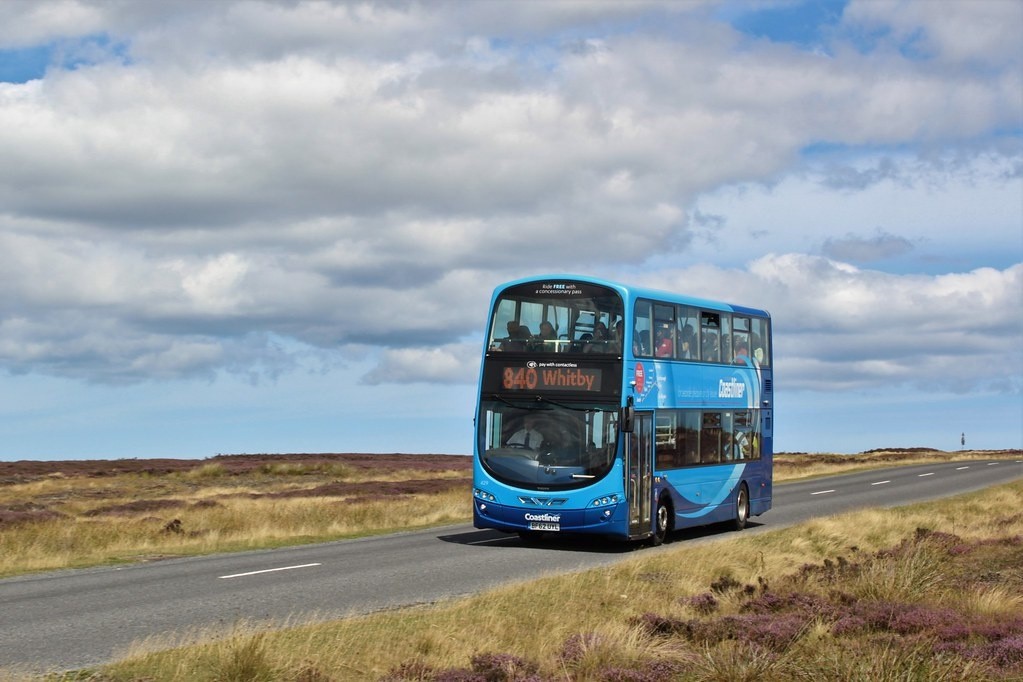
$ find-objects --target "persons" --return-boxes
[505,414,543,454]
[673,428,760,464]
[495,321,562,353]
[567,324,763,365]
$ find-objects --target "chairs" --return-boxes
[519,326,765,366]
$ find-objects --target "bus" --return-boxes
[473,274,773,547]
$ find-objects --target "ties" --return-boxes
[525,433,530,447]
